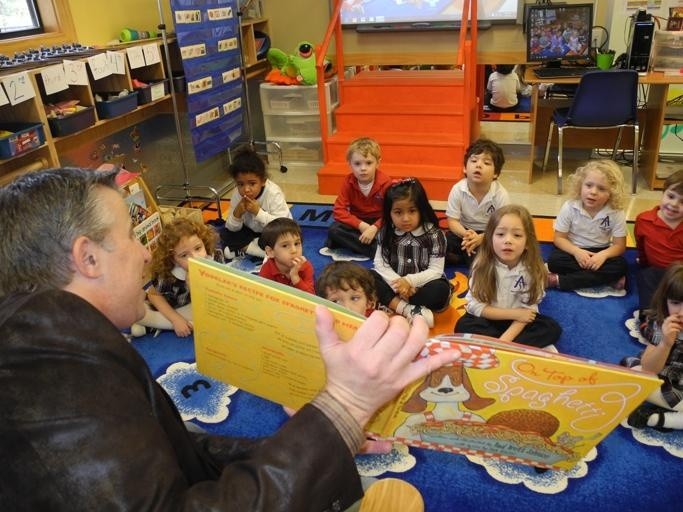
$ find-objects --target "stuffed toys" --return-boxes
[265,41,318,86]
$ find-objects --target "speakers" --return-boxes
[634,7,650,24]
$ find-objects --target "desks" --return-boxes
[522,63,677,192]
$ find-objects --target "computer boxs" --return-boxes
[626,18,655,76]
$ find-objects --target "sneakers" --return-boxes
[223,244,245,261]
[129,322,159,338]
[408,304,435,331]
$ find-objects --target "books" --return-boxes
[188,256,663,471]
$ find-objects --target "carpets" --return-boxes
[117,198,682,512]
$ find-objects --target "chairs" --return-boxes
[543,66,641,196]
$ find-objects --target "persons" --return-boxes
[443,139,508,269]
[634,170,683,316]
[220,146,293,264]
[544,159,629,288]
[328,137,400,258]
[0,166,460,512]
[622,261,682,434]
[371,174,454,330]
[257,217,313,294]
[132,218,220,336]
[486,63,520,112]
[455,204,561,353]
[315,260,376,318]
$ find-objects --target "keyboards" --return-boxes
[531,67,604,78]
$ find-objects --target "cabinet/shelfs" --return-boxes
[1,20,281,208]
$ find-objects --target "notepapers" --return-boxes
[88,53,113,81]
[106,50,126,75]
[62,59,89,86]
[41,64,69,95]
[0,85,9,107]
[151,83,166,101]
[142,43,161,66]
[126,46,146,69]
[2,72,36,107]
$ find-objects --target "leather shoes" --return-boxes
[614,276,626,291]
[626,405,677,432]
[545,272,557,288]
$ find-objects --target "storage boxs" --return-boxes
[0,119,45,159]
[96,90,139,120]
[264,142,329,166]
[46,106,97,136]
[171,69,186,91]
[139,79,169,104]
[261,116,336,142]
[260,80,336,113]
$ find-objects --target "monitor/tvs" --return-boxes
[525,3,595,65]
[327,1,521,30]
